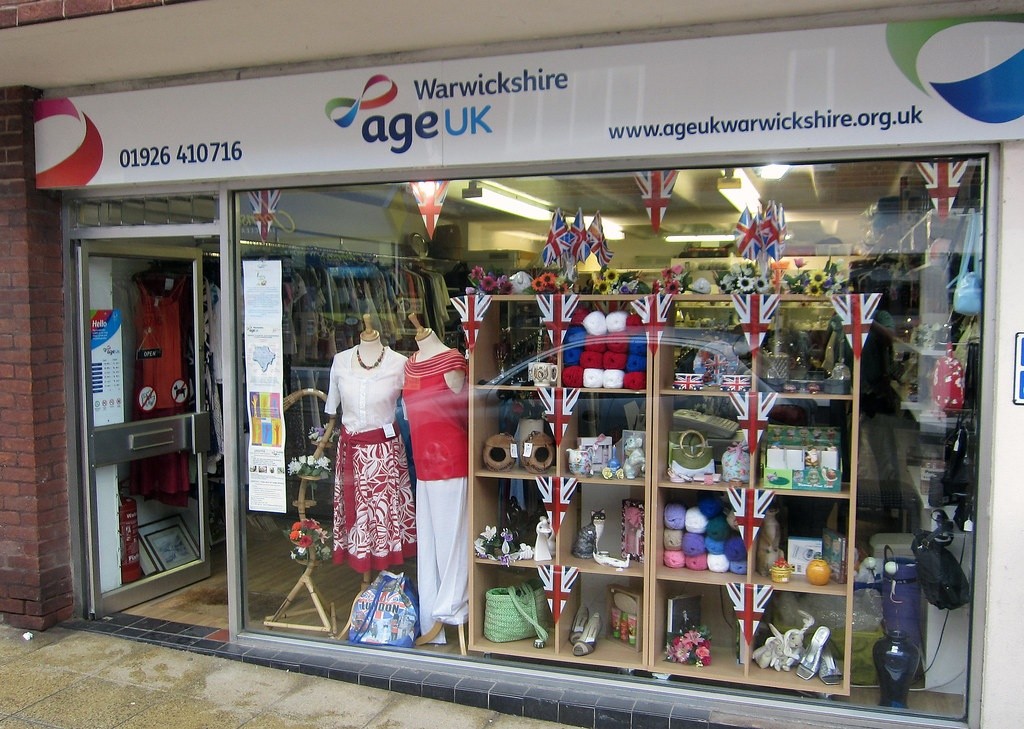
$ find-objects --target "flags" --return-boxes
[542,206,615,269]
[734,205,787,261]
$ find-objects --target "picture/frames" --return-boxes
[136,515,201,573]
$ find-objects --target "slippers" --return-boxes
[819,646,843,685]
[796,626,830,680]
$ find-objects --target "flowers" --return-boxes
[286,520,328,547]
[465,260,856,297]
[667,625,715,667]
[306,423,342,443]
[287,455,332,478]
[290,544,333,562]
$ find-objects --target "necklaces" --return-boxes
[357,346,384,370]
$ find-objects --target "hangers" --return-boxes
[242,238,427,274]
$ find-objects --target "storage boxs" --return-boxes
[761,424,843,493]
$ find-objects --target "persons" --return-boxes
[820,273,903,537]
[325,330,417,572]
[401,328,469,644]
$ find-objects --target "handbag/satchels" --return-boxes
[934,343,963,418]
[911,509,971,610]
[942,420,976,505]
[953,212,982,316]
[484,580,549,643]
[669,428,749,486]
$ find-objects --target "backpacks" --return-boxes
[350,570,420,648]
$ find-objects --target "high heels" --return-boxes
[569,606,589,647]
[572,613,602,656]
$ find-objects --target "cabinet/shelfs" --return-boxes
[460,293,866,696]
[873,197,973,694]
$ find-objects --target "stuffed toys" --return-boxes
[752,610,816,671]
